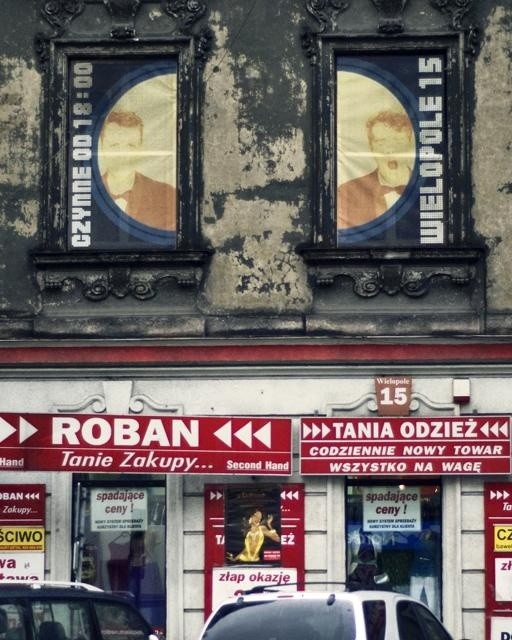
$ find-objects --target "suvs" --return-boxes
[200,581,457,640]
[0,579,160,640]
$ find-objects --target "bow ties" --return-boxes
[379,185,407,196]
[110,190,132,203]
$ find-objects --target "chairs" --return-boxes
[38,620,66,640]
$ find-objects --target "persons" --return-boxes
[97,110,175,233]
[337,107,415,236]
[349,528,385,581]
[410,527,438,617]
[226,510,280,565]
